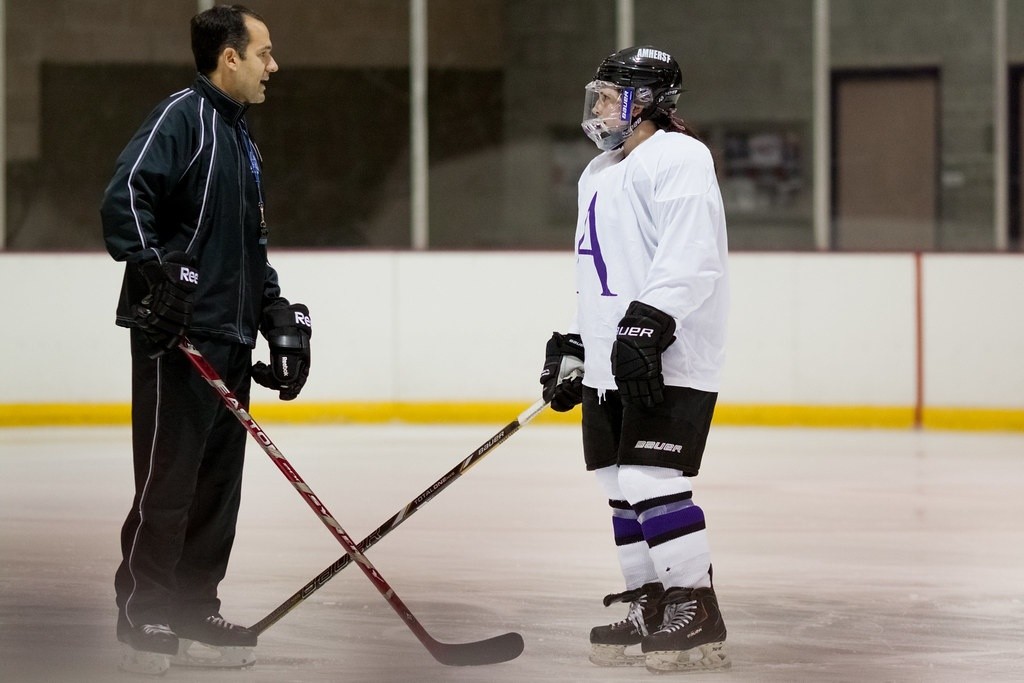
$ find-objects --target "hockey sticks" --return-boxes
[239,366,587,639]
[171,329,528,669]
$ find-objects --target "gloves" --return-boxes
[540,332,584,414]
[131,251,200,359]
[611,301,678,409]
[252,303,312,400]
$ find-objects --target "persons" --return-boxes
[99,7,311,675]
[538,45,732,670]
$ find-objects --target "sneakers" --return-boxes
[170,613,257,668]
[116,623,179,675]
[588,582,666,666]
[641,563,731,673]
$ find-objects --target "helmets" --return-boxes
[581,45,683,150]
